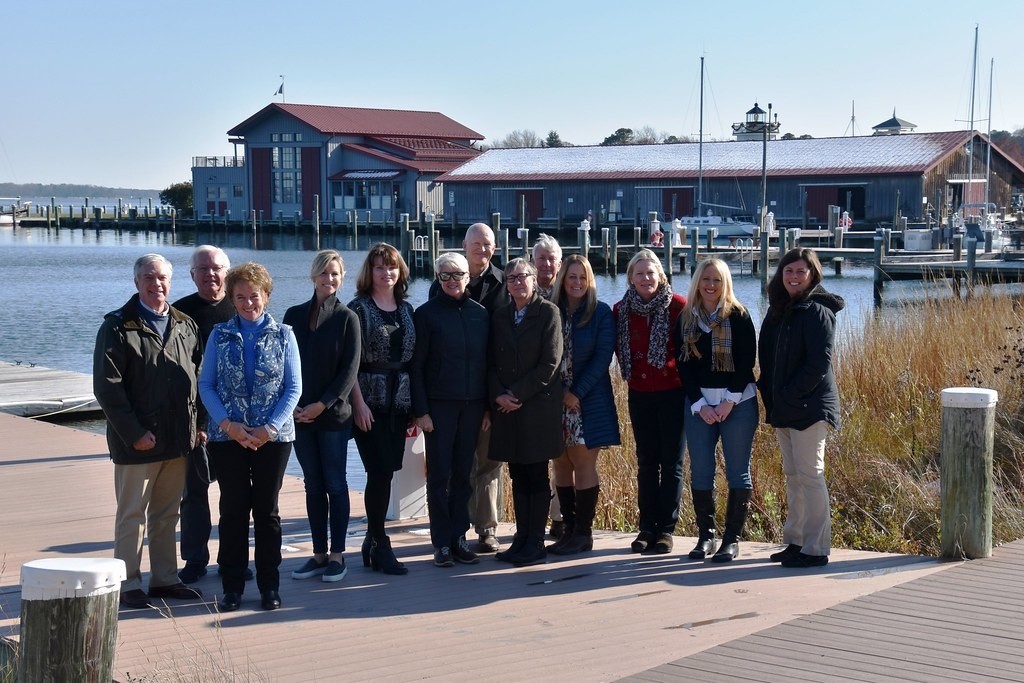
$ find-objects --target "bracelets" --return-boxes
[225,422,231,433]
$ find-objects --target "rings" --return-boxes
[718,416,721,418]
[707,419,710,422]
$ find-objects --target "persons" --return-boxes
[674,259,761,562]
[757,247,844,568]
[197,261,302,612]
[613,250,687,554]
[170,245,254,582]
[93,254,207,608]
[533,234,565,537]
[487,257,566,566]
[346,244,415,575]
[428,222,511,552]
[413,252,494,566]
[545,255,621,555]
[283,250,362,581]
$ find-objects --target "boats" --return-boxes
[0,194,32,225]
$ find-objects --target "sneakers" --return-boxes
[452,535,480,564]
[434,544,455,566]
[291,554,328,579]
[782,552,828,568]
[321,556,347,582]
[771,544,803,561]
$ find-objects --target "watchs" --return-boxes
[264,424,278,441]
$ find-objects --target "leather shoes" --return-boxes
[259,590,281,610]
[120,588,152,608]
[479,533,499,551]
[148,582,201,598]
[221,592,241,611]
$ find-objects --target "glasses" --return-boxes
[505,273,534,283]
[438,272,467,282]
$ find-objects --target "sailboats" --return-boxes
[942,22,1016,252]
[661,55,760,239]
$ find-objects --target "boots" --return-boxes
[496,481,548,566]
[556,485,600,554]
[688,490,717,559]
[546,486,576,553]
[711,488,752,563]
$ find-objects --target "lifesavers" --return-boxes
[122,207,124,212]
[840,217,852,227]
[651,233,665,246]
[25,203,29,210]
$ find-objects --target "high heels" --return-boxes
[370,536,408,574]
[361,530,404,567]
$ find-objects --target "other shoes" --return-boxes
[655,532,673,553]
[631,531,654,552]
[218,565,253,580]
[177,563,208,584]
[550,520,567,538]
